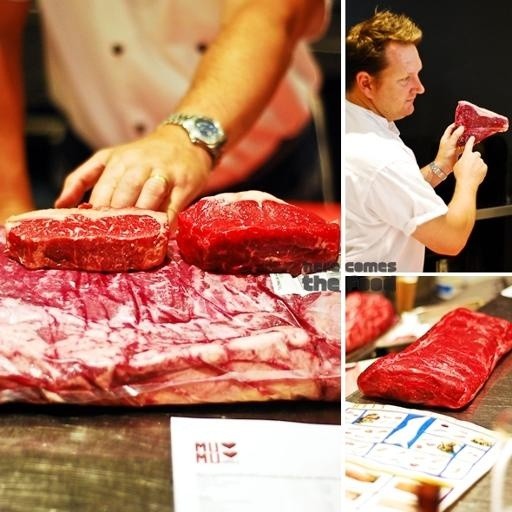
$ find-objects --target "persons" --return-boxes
[2,1,338,226]
[344,6,490,276]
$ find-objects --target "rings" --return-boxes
[150,174,170,192]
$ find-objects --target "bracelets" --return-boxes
[429,161,448,182]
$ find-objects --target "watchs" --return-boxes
[163,113,227,169]
